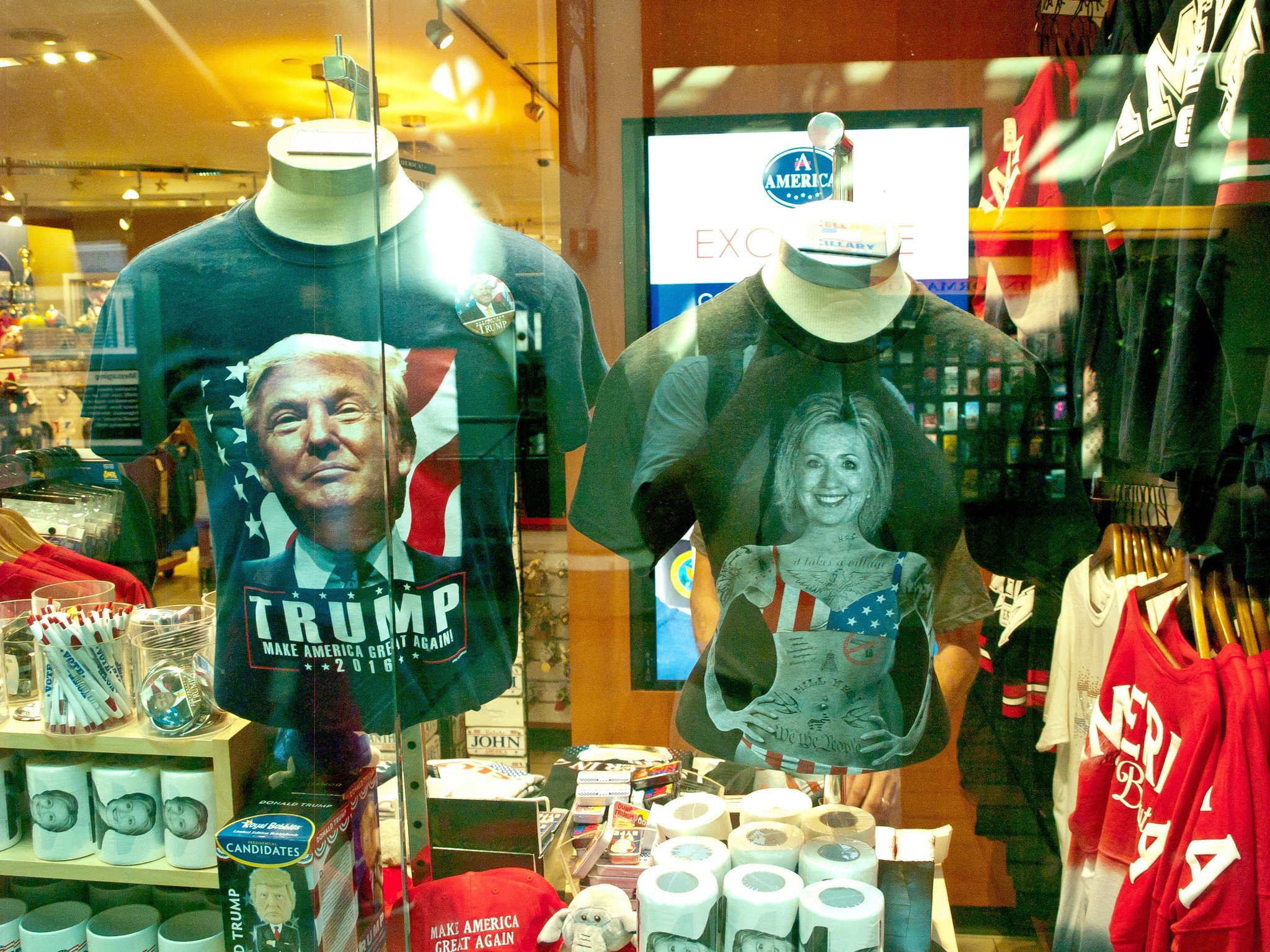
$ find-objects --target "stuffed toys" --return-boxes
[537,884,637,952]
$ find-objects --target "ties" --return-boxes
[331,561,376,590]
[177,692,184,697]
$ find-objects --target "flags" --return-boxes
[144,673,185,726]
[200,348,462,561]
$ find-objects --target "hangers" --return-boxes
[1089,482,1270,667]
[0,446,139,596]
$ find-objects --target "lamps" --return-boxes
[523,86,544,122]
[425,0,454,50]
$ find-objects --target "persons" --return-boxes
[732,929,795,952]
[689,520,995,825]
[83,117,611,886]
[798,926,828,952]
[30,790,78,832]
[149,673,190,720]
[569,199,1099,816]
[247,868,301,952]
[226,332,465,675]
[646,903,718,952]
[632,325,932,690]
[92,780,156,850]
[161,796,207,840]
[703,391,936,778]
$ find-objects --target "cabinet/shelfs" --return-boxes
[0,707,277,952]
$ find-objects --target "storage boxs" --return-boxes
[215,767,387,952]
[571,761,682,910]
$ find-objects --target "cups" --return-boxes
[0,580,237,739]
[0,875,224,952]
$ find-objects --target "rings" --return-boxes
[882,798,890,806]
